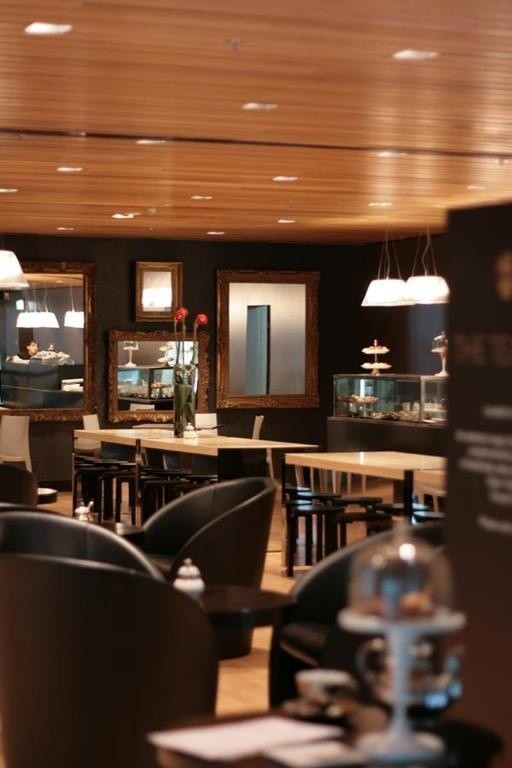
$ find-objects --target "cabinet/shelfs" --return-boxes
[327,373,452,455]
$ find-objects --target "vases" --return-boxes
[173,366,196,438]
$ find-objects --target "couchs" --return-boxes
[1,361,84,406]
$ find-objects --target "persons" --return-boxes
[18,339,40,360]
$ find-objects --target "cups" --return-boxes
[296,668,353,707]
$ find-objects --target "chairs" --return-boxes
[0,416,512,768]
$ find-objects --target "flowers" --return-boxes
[172,307,208,365]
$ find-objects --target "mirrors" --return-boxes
[134,261,184,324]
[107,328,212,424]
[215,267,321,410]
[0,261,98,423]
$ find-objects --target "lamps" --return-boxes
[360,227,413,308]
[0,248,30,291]
[404,223,450,305]
[16,281,84,329]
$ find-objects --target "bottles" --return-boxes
[182,423,198,440]
[74,500,94,524]
[171,558,205,601]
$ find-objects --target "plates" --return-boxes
[278,696,356,720]
